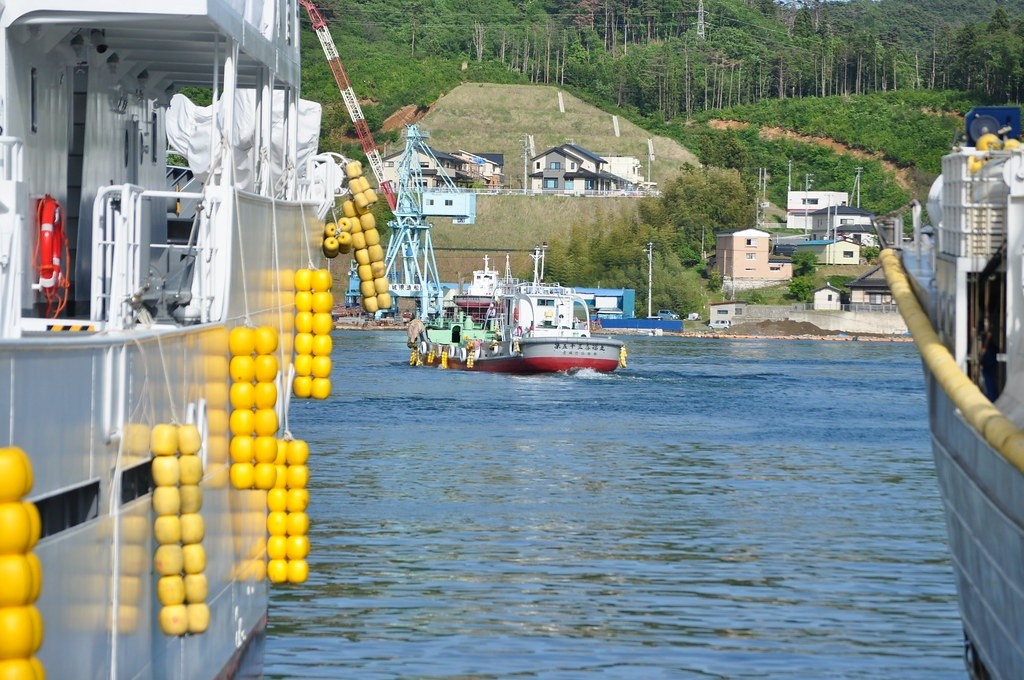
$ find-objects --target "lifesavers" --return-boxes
[419,341,427,355]
[489,341,499,355]
[448,345,455,358]
[435,344,442,358]
[472,341,480,362]
[509,340,519,358]
[459,348,467,362]
[35,194,64,298]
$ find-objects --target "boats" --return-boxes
[1,0,393,680]
[406,247,627,372]
[873,106,1024,680]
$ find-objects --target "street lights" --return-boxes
[643,243,653,319]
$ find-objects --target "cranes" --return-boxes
[301,0,461,325]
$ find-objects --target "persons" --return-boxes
[485,302,496,330]
[839,232,849,241]
[402,309,412,325]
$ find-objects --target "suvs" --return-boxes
[658,310,681,319]
[708,320,731,329]
